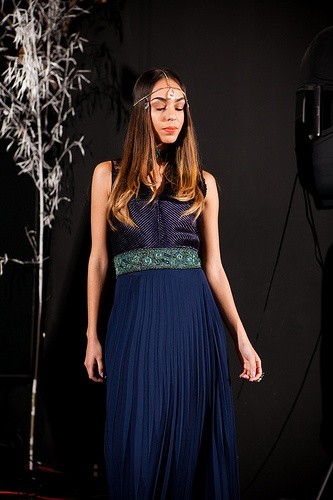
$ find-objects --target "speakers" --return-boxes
[296,25,333,210]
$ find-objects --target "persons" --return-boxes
[84,68,266,500]
